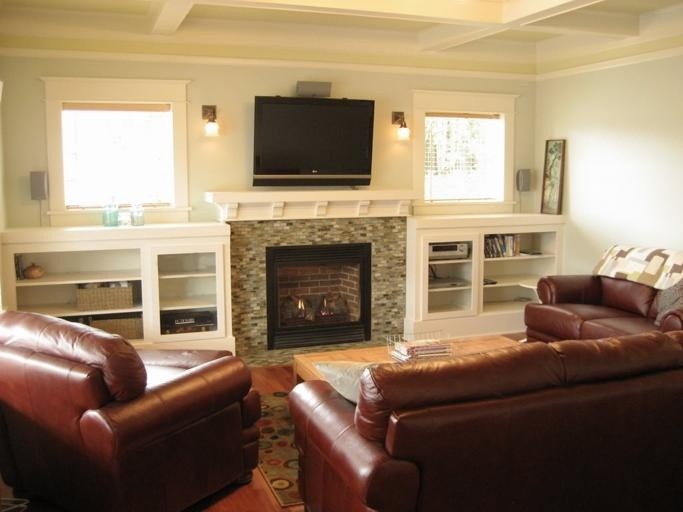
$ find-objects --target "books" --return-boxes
[483,235,520,257]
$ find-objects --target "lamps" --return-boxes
[201,105,218,138]
[393,111,410,141]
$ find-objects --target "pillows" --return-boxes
[648,290,663,319]
[547,330,682,387]
[354,341,565,443]
[599,276,659,318]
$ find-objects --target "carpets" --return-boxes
[255,391,305,512]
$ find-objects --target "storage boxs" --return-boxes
[75,282,135,310]
[88,316,143,340]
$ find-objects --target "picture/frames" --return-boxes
[540,140,565,216]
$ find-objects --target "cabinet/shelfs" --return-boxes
[0,223,236,353]
[403,213,564,342]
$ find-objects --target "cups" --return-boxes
[130,206,145,226]
[101,204,119,227]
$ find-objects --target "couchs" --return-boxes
[525,245,683,341]
[289,329,682,510]
[0,310,263,511]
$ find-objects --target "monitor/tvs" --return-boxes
[252,95,375,186]
